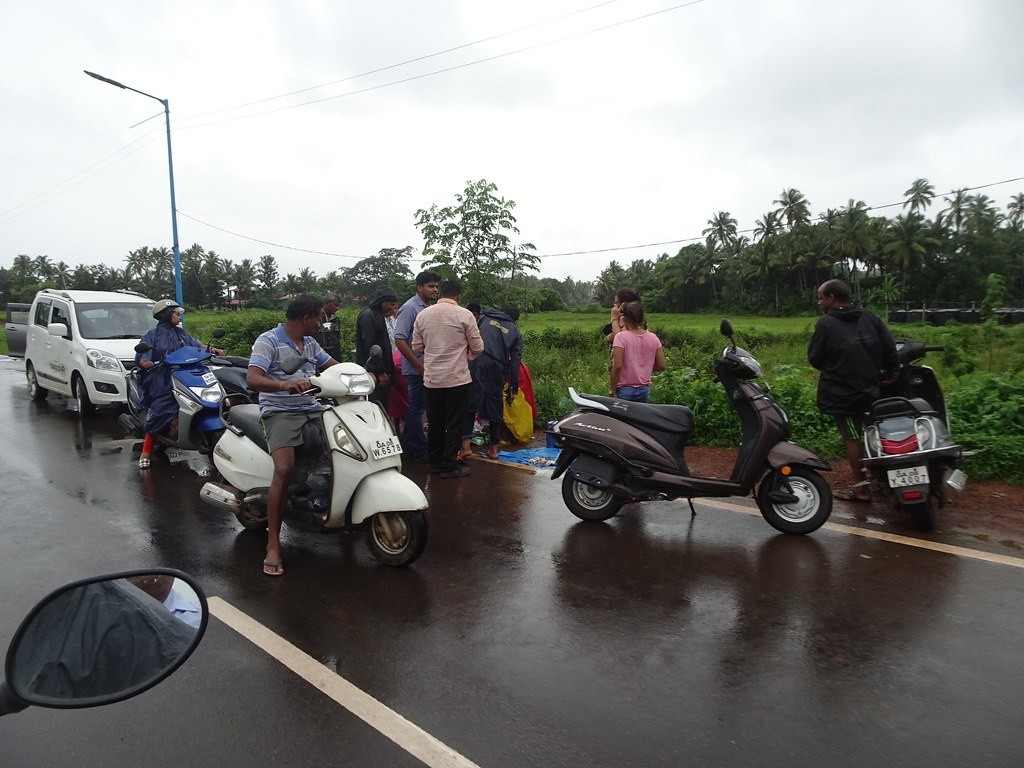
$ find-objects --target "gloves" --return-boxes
[505,389,514,406]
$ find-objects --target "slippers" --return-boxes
[264,559,283,575]
[832,487,871,504]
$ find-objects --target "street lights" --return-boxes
[82,70,183,330]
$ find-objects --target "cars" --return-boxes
[5,302,108,359]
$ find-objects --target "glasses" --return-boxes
[613,301,621,306]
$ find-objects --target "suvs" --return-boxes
[26,289,182,428]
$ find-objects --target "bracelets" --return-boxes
[280,380,290,391]
[611,318,619,322]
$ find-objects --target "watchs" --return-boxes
[608,389,616,394]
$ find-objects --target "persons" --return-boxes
[134,298,229,470]
[604,288,666,403]
[246,293,344,574]
[807,279,901,502]
[355,270,523,478]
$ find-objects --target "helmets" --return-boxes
[152,299,180,318]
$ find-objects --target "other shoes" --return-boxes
[440,465,472,478]
[431,459,465,474]
[139,453,150,468]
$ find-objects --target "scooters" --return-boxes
[537,318,833,537]
[853,338,970,533]
[122,327,233,469]
[200,344,429,569]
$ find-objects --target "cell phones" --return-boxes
[620,303,626,314]
